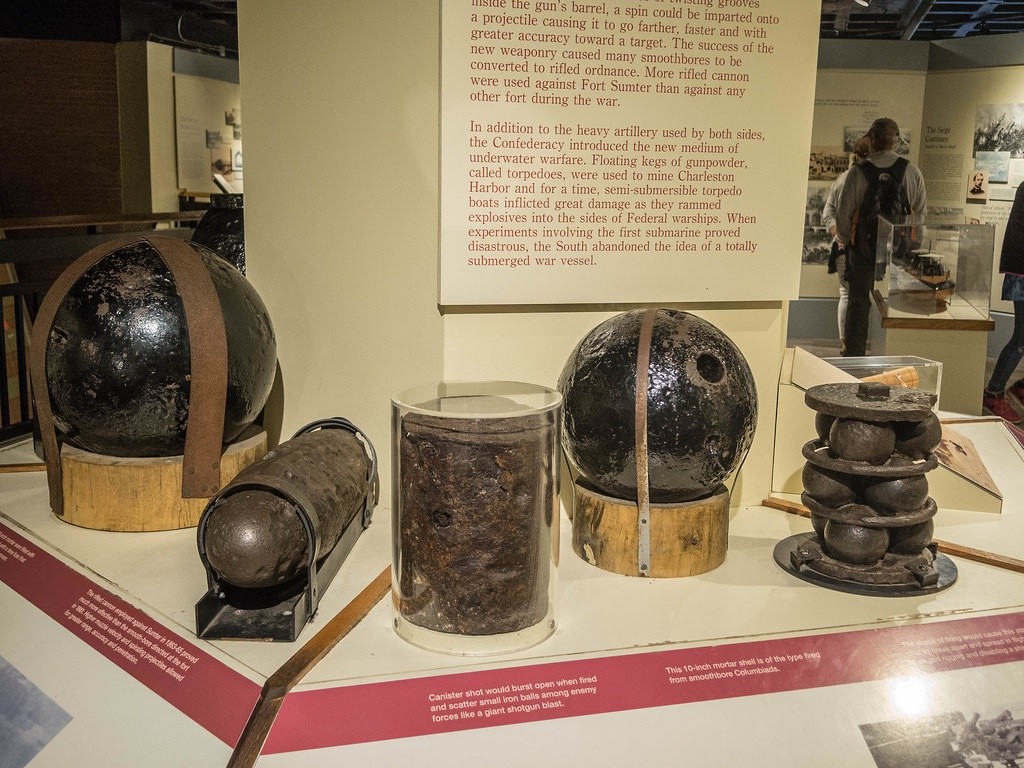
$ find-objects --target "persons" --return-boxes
[984,180,1024,424]
[822,118,928,358]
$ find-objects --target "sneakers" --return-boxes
[983,388,1022,424]
[1007,380,1024,412]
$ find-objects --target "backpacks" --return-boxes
[854,157,912,270]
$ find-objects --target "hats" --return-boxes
[861,116,899,140]
